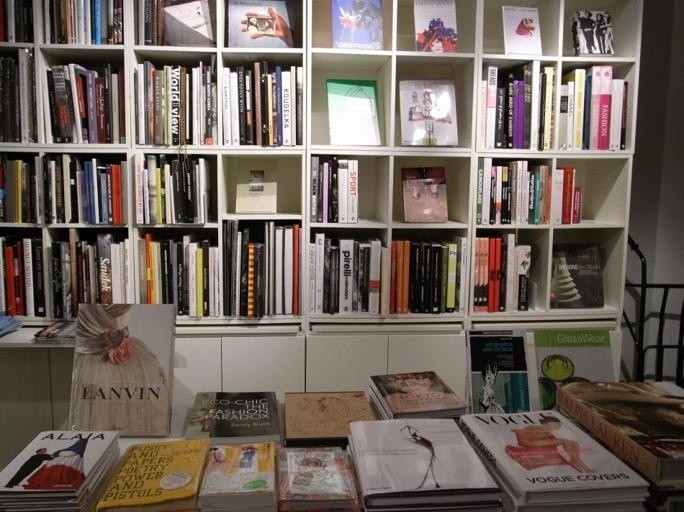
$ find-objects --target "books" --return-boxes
[329,0,617,59]
[327,66,629,152]
[311,157,581,226]
[276,372,503,512]
[68,303,177,437]
[1,390,279,512]
[462,328,684,512]
[309,233,604,315]
[1,0,304,319]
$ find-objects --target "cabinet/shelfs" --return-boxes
[0,344,50,463]
[0,1,308,327]
[390,331,467,408]
[305,332,390,398]
[50,349,74,434]
[305,0,643,317]
[172,335,223,408]
[222,333,305,401]
